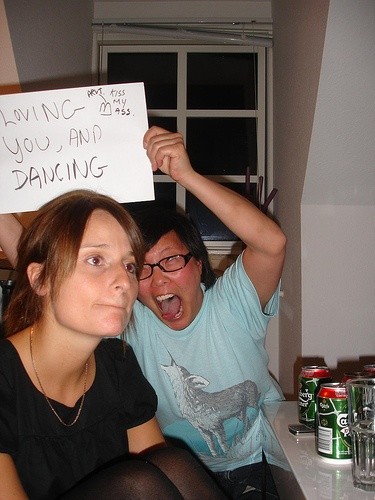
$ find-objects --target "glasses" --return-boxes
[137,251,193,281]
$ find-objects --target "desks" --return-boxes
[260,400,375,500]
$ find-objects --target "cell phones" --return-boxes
[288,425,316,435]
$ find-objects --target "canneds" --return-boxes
[314,383,360,460]
[297,365,330,427]
[340,364,375,386]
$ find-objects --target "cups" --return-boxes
[347,379,375,492]
